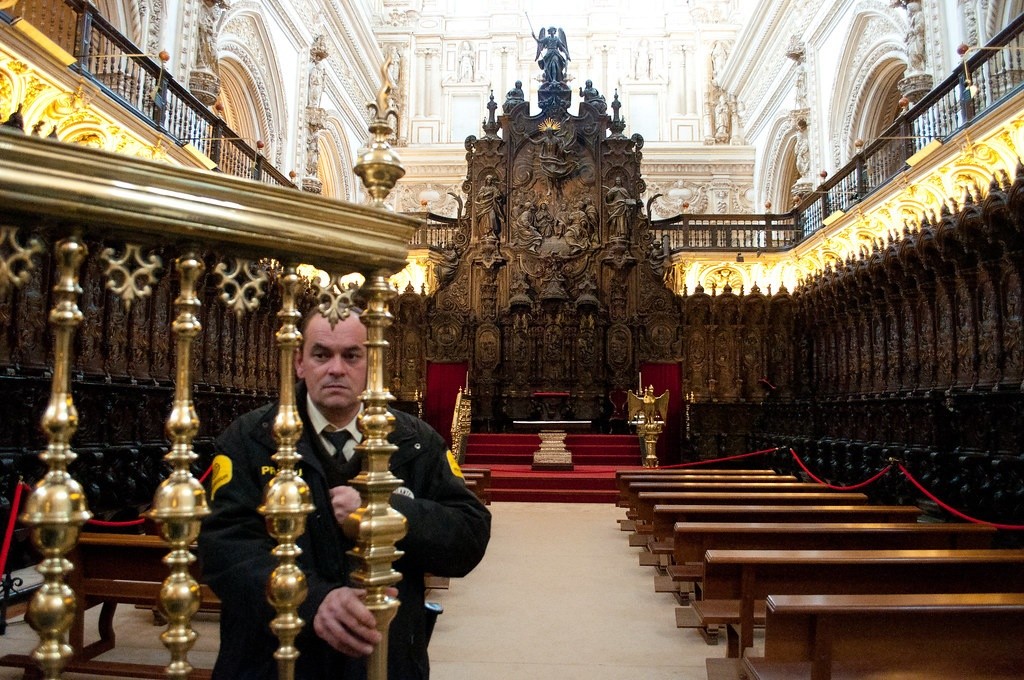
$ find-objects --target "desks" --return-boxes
[512,420,592,472]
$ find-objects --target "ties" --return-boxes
[321,430,353,469]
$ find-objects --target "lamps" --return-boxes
[962,85,978,101]
[737,253,744,262]
[149,86,163,111]
[860,168,873,181]
[757,250,763,258]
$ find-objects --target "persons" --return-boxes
[713,95,729,134]
[475,175,504,239]
[554,201,571,238]
[563,200,588,254]
[185,301,492,680]
[441,243,459,281]
[517,201,541,252]
[605,177,633,240]
[308,61,324,107]
[711,43,726,80]
[632,39,652,81]
[198,2,221,68]
[537,202,553,238]
[904,1,926,73]
[584,197,599,249]
[794,131,810,178]
[456,42,474,83]
[579,79,607,113]
[649,240,667,277]
[522,127,567,199]
[531,26,571,82]
[502,80,524,113]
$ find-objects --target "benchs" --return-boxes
[0,467,492,680]
[615,468,1024,680]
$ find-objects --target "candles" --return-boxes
[638,371,642,391]
[465,371,469,389]
